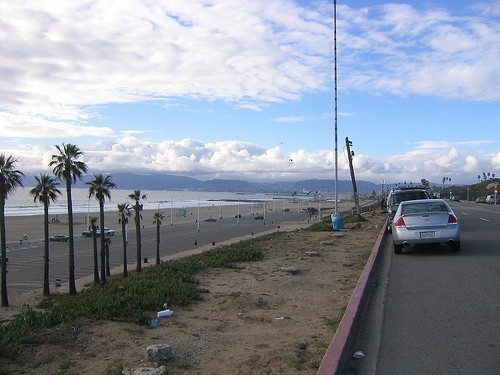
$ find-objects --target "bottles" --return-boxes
[157,309,173,317]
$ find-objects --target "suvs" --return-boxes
[385,187,435,234]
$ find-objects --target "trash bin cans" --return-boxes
[331,213,346,230]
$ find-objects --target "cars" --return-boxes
[81,226,115,238]
[254,215,264,220]
[204,217,216,222]
[48,234,69,242]
[485,194,500,205]
[452,196,460,202]
[475,196,487,203]
[390,198,460,254]
